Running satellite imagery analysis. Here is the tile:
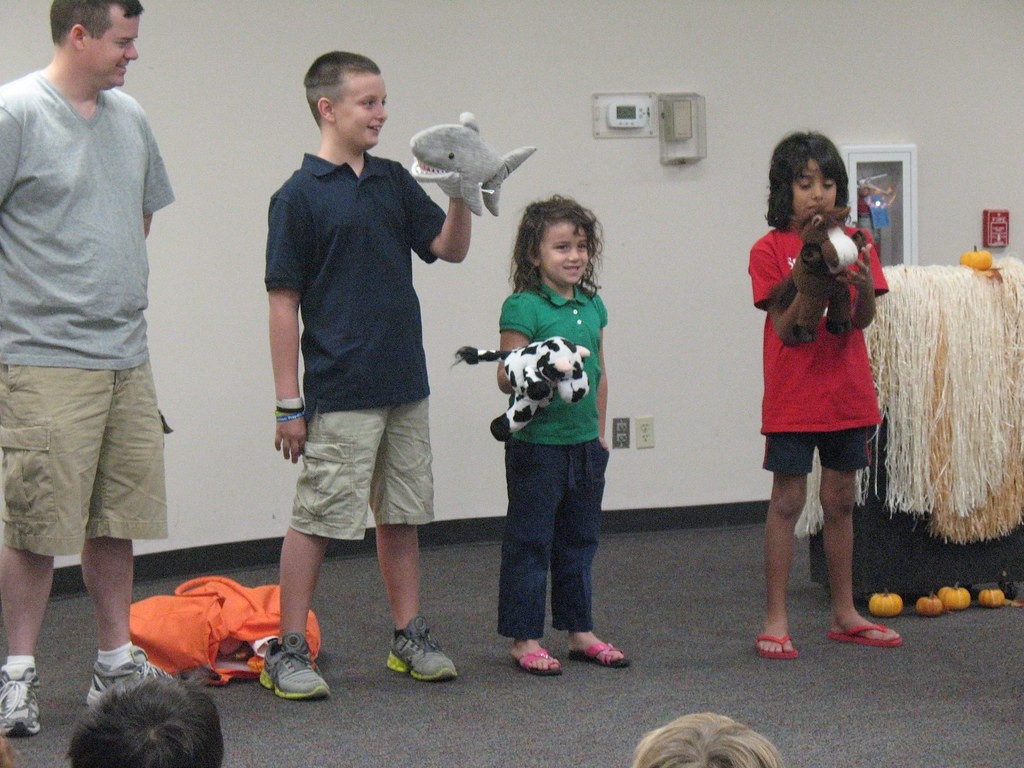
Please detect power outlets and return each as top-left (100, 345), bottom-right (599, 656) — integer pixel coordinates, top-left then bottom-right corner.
top-left (612, 418), bottom-right (631, 448)
top-left (634, 416), bottom-right (655, 447)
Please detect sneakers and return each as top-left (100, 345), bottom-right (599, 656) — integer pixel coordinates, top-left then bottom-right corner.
top-left (1, 666), bottom-right (46, 736)
top-left (262, 633), bottom-right (330, 700)
top-left (85, 657), bottom-right (172, 708)
top-left (387, 616), bottom-right (458, 680)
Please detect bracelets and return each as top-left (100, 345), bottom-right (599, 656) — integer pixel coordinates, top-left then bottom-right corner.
top-left (275, 396), bottom-right (307, 422)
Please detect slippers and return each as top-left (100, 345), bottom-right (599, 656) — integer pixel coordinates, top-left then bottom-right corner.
top-left (826, 622), bottom-right (903, 647)
top-left (569, 640), bottom-right (625, 665)
top-left (753, 634), bottom-right (801, 659)
top-left (511, 643), bottom-right (562, 674)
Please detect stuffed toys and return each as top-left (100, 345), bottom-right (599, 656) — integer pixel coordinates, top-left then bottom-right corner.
top-left (769, 205), bottom-right (868, 342)
top-left (450, 336), bottom-right (591, 442)
top-left (409, 111), bottom-right (538, 216)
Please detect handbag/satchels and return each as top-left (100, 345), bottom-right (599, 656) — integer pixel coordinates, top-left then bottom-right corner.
top-left (129, 573), bottom-right (323, 686)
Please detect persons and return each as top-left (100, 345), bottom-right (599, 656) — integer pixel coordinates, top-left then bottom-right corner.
top-left (747, 130), bottom-right (904, 659)
top-left (67, 671), bottom-right (224, 768)
top-left (258, 50), bottom-right (472, 700)
top-left (0, 0), bottom-right (177, 738)
top-left (632, 712), bottom-right (785, 768)
top-left (496, 194), bottom-right (629, 677)
